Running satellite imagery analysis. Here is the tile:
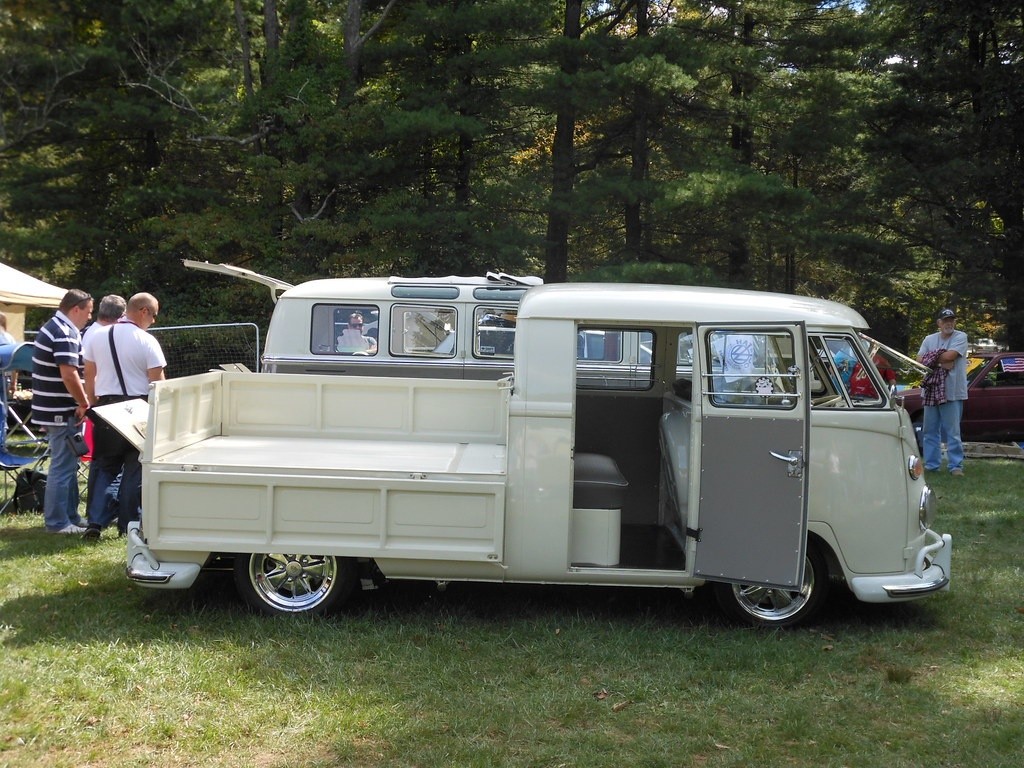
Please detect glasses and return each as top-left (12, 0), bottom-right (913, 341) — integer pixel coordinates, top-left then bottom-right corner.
top-left (73, 294), bottom-right (91, 307)
top-left (140, 307), bottom-right (158, 323)
top-left (349, 323), bottom-right (363, 328)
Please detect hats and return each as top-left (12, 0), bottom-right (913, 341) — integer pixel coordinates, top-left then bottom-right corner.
top-left (939, 309), bottom-right (956, 320)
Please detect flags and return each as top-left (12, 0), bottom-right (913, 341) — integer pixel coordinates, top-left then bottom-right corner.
top-left (1001, 357), bottom-right (1024, 372)
top-left (714, 335), bottom-right (754, 382)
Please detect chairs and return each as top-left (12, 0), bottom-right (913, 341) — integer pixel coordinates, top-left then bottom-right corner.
top-left (0, 342), bottom-right (94, 515)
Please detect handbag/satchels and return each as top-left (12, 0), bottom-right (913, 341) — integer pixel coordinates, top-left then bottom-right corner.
top-left (64, 417), bottom-right (89, 457)
top-left (13, 469), bottom-right (48, 513)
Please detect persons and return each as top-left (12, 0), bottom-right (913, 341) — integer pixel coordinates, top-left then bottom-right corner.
top-left (0, 314), bottom-right (19, 452)
top-left (338, 313), bottom-right (377, 353)
top-left (850, 352), bottom-right (896, 397)
top-left (30, 288), bottom-right (94, 533)
top-left (914, 309), bottom-right (969, 476)
top-left (80, 293), bottom-right (168, 539)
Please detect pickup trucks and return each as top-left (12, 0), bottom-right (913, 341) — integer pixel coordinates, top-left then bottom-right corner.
top-left (92, 283), bottom-right (953, 630)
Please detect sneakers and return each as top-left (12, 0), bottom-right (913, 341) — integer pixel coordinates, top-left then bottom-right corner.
top-left (47, 524), bottom-right (88, 534)
top-left (77, 517), bottom-right (89, 527)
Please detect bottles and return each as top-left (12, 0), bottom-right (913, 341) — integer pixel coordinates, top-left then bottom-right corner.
top-left (17, 383), bottom-right (22, 391)
top-left (7, 379), bottom-right (10, 387)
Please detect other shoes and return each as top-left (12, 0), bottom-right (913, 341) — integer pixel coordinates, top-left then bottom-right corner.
top-left (79, 522), bottom-right (102, 539)
top-left (117, 526), bottom-right (127, 537)
top-left (951, 469), bottom-right (964, 476)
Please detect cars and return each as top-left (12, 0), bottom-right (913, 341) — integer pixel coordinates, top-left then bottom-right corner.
top-left (895, 352), bottom-right (1024, 443)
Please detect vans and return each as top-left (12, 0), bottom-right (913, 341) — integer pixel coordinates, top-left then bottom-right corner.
top-left (183, 259), bottom-right (654, 381)
top-left (371, 307), bottom-right (652, 364)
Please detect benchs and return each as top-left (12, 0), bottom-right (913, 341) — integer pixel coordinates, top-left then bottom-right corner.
top-left (571, 451), bottom-right (631, 566)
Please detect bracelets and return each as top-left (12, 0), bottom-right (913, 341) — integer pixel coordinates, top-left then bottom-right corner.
top-left (11, 381), bottom-right (16, 384)
